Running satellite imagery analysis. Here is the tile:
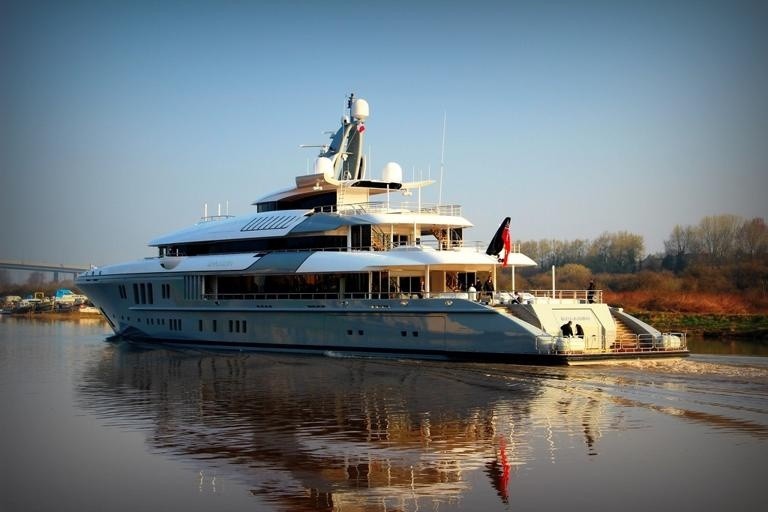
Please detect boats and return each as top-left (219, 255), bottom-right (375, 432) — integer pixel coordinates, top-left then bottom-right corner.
top-left (76, 90), bottom-right (691, 366)
top-left (0, 289), bottom-right (102, 314)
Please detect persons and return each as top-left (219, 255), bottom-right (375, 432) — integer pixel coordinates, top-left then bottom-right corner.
top-left (561, 320), bottom-right (573, 338)
top-left (473, 277), bottom-right (482, 299)
top-left (586, 279), bottom-right (594, 303)
top-left (576, 324), bottom-right (584, 339)
top-left (512, 291), bottom-right (520, 304)
top-left (483, 276), bottom-right (494, 299)
